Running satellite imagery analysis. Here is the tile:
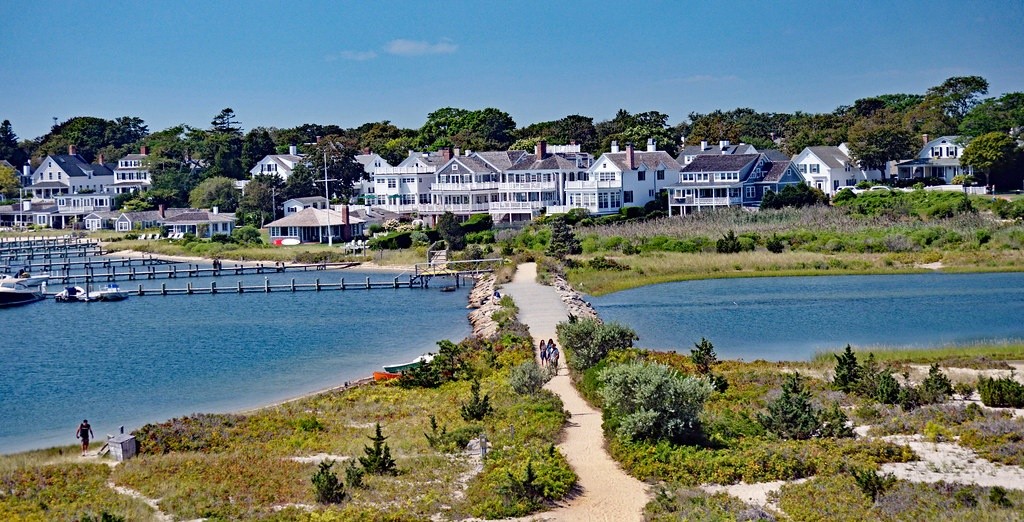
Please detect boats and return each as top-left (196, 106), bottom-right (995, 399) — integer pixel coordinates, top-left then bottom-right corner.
top-left (0, 279), bottom-right (46, 307)
top-left (55, 286), bottom-right (85, 302)
top-left (0, 274), bottom-right (50, 286)
top-left (99, 288), bottom-right (129, 300)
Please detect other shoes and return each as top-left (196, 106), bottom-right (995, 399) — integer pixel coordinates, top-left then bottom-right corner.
top-left (82, 453), bottom-right (88, 456)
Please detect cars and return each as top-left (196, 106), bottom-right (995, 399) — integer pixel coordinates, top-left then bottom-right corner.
top-left (870, 186), bottom-right (891, 191)
top-left (842, 186), bottom-right (866, 194)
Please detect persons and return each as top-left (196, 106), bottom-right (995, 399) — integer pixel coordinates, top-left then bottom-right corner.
top-left (540, 339), bottom-right (559, 376)
top-left (76, 419), bottom-right (94, 456)
top-left (493, 290), bottom-right (501, 298)
